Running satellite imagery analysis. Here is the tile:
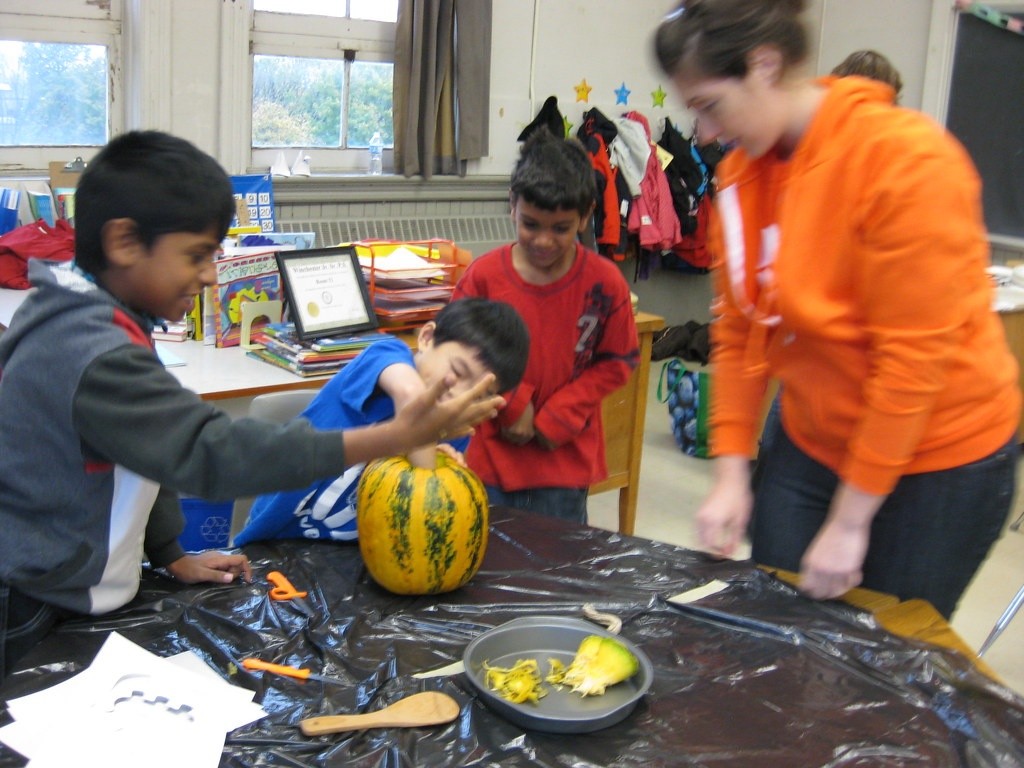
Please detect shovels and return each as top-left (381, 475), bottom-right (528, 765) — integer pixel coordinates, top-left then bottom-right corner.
top-left (300, 692), bottom-right (460, 736)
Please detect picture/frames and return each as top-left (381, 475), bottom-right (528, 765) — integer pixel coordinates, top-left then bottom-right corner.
top-left (273, 244), bottom-right (380, 339)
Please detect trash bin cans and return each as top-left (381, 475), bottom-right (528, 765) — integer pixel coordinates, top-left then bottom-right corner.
top-left (178, 496), bottom-right (235, 554)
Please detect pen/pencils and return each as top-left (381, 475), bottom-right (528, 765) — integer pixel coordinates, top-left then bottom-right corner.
top-left (164, 363), bottom-right (187, 368)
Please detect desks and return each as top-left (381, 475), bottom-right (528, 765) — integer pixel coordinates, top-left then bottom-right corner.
top-left (0, 307), bottom-right (1024, 765)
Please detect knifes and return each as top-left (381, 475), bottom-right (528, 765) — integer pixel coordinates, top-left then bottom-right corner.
top-left (243, 660), bottom-right (357, 688)
top-left (267, 571), bottom-right (314, 619)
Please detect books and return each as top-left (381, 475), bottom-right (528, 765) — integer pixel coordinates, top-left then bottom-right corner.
top-left (152, 244), bottom-right (450, 378)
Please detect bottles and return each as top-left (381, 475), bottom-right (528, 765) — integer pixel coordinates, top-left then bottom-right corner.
top-left (368, 132), bottom-right (383, 175)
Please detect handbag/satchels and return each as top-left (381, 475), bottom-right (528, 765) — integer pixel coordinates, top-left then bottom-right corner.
top-left (656, 359), bottom-right (721, 459)
top-left (0, 219), bottom-right (75, 291)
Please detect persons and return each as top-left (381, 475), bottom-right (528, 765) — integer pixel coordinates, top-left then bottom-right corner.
top-left (652, 0), bottom-right (1023, 624)
top-left (0, 129), bottom-right (507, 688)
top-left (232, 298), bottom-right (530, 546)
top-left (450, 138), bottom-right (640, 525)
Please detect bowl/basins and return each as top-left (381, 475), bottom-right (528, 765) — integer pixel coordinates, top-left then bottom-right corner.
top-left (463, 615), bottom-right (653, 732)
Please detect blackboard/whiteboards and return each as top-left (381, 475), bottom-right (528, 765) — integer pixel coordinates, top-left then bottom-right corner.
top-left (920, 0), bottom-right (1024, 254)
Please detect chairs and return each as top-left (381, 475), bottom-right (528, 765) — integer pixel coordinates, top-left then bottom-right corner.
top-left (231, 384), bottom-right (324, 550)
